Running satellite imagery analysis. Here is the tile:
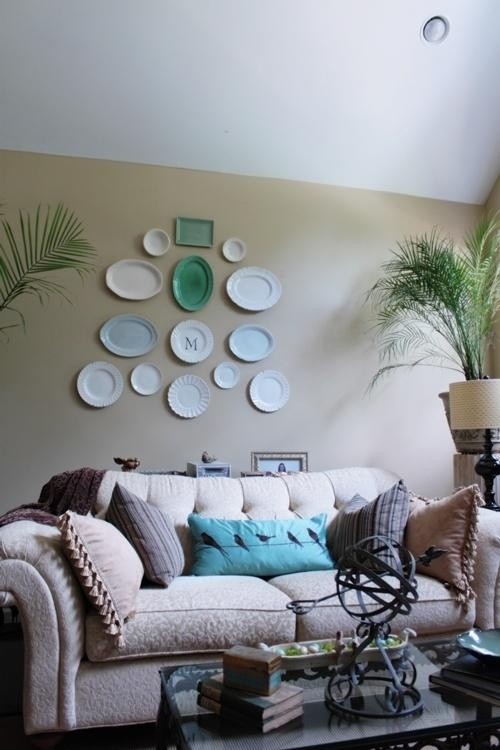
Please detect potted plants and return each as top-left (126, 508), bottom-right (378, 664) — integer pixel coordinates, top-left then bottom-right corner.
top-left (360, 208), bottom-right (500, 452)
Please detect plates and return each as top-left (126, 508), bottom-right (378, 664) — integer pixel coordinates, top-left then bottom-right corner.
top-left (169, 318), bottom-right (214, 364)
top-left (167, 374), bottom-right (210, 418)
top-left (99, 313), bottom-right (160, 358)
top-left (213, 361), bottom-right (241, 390)
top-left (130, 362), bottom-right (164, 397)
top-left (105, 258), bottom-right (164, 302)
top-left (228, 323), bottom-right (276, 363)
top-left (225, 265), bottom-right (283, 312)
top-left (249, 369), bottom-right (290, 413)
top-left (76, 360), bottom-right (124, 408)
top-left (175, 216), bottom-right (215, 248)
top-left (172, 254), bottom-right (215, 312)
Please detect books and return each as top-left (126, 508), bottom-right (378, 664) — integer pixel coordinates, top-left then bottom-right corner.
top-left (429, 655), bottom-right (500, 706)
top-left (197, 675), bottom-right (304, 734)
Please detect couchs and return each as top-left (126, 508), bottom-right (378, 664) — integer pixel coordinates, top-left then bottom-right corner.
top-left (0, 468), bottom-right (500, 737)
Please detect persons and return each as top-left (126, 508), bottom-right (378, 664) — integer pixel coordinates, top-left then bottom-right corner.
top-left (278, 463), bottom-right (286, 472)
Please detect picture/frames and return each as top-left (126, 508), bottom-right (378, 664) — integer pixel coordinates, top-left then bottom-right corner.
top-left (251, 451), bottom-right (309, 473)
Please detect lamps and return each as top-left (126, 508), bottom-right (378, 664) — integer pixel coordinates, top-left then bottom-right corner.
top-left (424, 19), bottom-right (445, 42)
top-left (449, 378), bottom-right (500, 511)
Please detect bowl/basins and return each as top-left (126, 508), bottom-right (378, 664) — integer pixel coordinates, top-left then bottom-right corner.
top-left (143, 228), bottom-right (171, 257)
top-left (456, 627), bottom-right (500, 666)
top-left (222, 238), bottom-right (247, 262)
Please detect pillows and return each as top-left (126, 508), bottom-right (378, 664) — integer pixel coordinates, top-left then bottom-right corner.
top-left (59, 511), bottom-right (146, 649)
top-left (187, 513), bottom-right (336, 575)
top-left (327, 480), bottom-right (410, 575)
top-left (106, 482), bottom-right (186, 588)
top-left (405, 484), bottom-right (486, 613)
